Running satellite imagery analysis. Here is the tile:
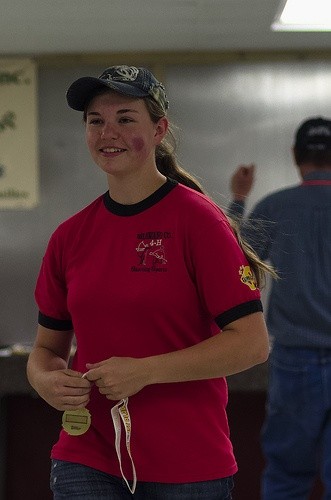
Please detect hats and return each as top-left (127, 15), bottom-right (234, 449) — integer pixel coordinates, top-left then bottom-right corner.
top-left (296, 118), bottom-right (330, 150)
top-left (65, 65), bottom-right (168, 112)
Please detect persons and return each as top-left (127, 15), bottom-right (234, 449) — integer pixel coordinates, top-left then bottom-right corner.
top-left (24, 66), bottom-right (273, 500)
top-left (222, 115), bottom-right (331, 500)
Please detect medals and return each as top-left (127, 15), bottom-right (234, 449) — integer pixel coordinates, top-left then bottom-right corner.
top-left (61, 402), bottom-right (92, 437)
